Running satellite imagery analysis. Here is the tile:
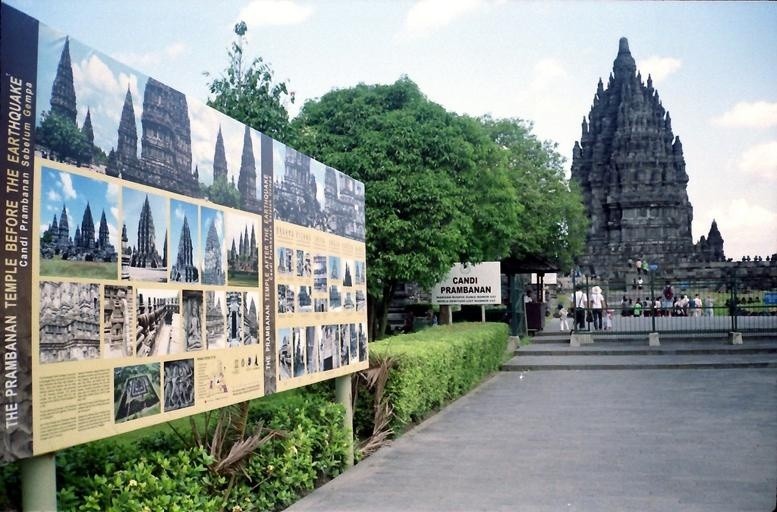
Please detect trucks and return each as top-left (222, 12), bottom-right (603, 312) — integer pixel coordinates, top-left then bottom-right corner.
top-left (385, 309), bottom-right (415, 334)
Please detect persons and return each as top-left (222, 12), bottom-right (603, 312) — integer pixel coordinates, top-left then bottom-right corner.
top-left (524, 258), bottom-right (715, 332)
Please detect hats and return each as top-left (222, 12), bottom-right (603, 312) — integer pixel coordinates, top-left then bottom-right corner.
top-left (576, 284), bottom-right (582, 289)
top-left (592, 286), bottom-right (603, 294)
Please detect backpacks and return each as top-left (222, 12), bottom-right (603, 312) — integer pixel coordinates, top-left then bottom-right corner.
top-left (664, 285), bottom-right (674, 300)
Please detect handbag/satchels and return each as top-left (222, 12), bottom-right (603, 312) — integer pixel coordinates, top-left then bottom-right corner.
top-left (575, 307), bottom-right (585, 322)
top-left (586, 311), bottom-right (595, 323)
